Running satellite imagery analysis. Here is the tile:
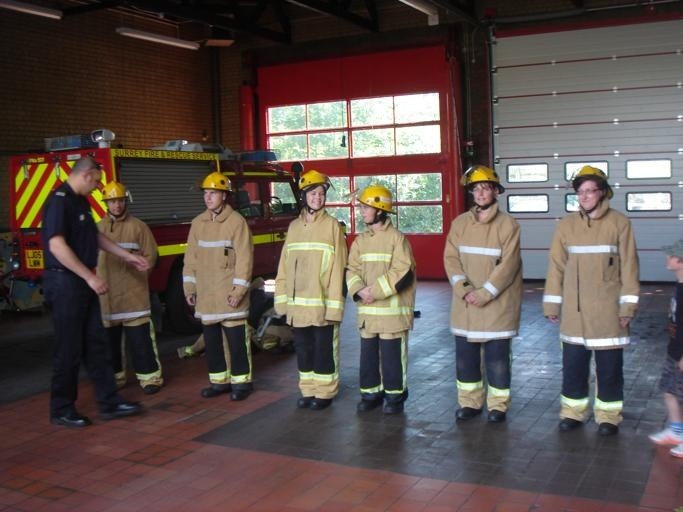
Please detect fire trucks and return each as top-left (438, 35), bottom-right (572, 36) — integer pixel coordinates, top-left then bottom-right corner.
top-left (6, 125), bottom-right (349, 338)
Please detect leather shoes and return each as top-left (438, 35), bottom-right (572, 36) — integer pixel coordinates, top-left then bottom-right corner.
top-left (49, 386), bottom-right (160, 427)
top-left (297, 397), bottom-right (314, 407)
top-left (382, 402), bottom-right (402, 414)
top-left (230, 389), bottom-right (249, 401)
top-left (358, 400), bottom-right (376, 411)
top-left (598, 423), bottom-right (618, 436)
top-left (309, 398), bottom-right (331, 409)
top-left (487, 410), bottom-right (504, 422)
top-left (455, 407), bottom-right (481, 420)
top-left (201, 384), bottom-right (231, 398)
top-left (558, 418), bottom-right (578, 430)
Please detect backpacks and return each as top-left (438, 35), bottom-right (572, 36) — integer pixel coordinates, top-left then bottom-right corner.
top-left (253, 307), bottom-right (296, 355)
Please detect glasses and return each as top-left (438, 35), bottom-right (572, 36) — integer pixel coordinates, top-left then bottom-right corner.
top-left (575, 188), bottom-right (601, 195)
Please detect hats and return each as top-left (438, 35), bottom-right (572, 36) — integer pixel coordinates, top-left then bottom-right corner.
top-left (661, 239), bottom-right (682, 258)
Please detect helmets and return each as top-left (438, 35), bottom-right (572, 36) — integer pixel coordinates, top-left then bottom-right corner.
top-left (356, 185), bottom-right (392, 213)
top-left (100, 181), bottom-right (128, 201)
top-left (298, 170), bottom-right (330, 190)
top-left (459, 165), bottom-right (504, 195)
top-left (565, 165), bottom-right (612, 199)
top-left (200, 172), bottom-right (232, 192)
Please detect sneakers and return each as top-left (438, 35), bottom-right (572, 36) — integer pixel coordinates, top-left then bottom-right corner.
top-left (669, 444), bottom-right (682, 459)
top-left (647, 429), bottom-right (682, 445)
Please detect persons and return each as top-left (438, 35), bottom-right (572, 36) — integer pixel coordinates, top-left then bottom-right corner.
top-left (442, 164), bottom-right (524, 424)
top-left (344, 184), bottom-right (416, 417)
top-left (95, 177), bottom-right (165, 395)
top-left (181, 171), bottom-right (254, 402)
top-left (272, 169), bottom-right (347, 410)
top-left (541, 164), bottom-right (641, 437)
top-left (40, 154), bottom-right (151, 428)
top-left (646, 239), bottom-right (682, 458)
